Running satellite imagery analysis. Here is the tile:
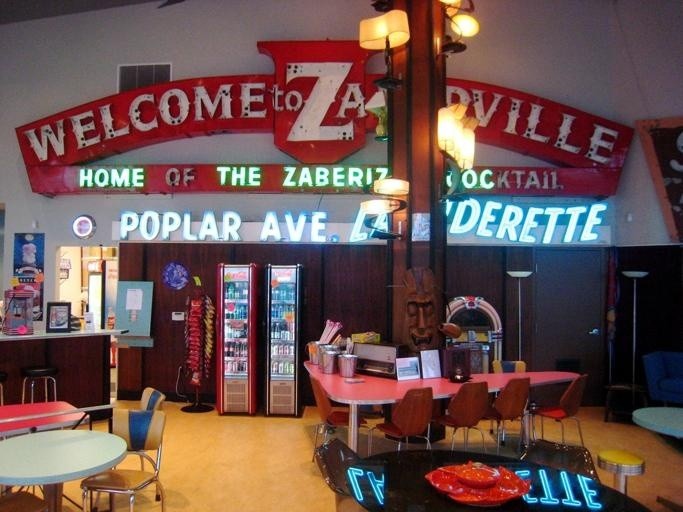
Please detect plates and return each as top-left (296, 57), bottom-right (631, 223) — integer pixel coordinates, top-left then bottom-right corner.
top-left (424, 461), bottom-right (530, 507)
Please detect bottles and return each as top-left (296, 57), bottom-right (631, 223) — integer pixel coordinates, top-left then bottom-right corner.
top-left (271, 321), bottom-right (294, 341)
top-left (224, 340), bottom-right (246, 357)
top-left (107, 308), bottom-right (114, 329)
top-left (270, 343), bottom-right (293, 355)
top-left (271, 287), bottom-right (295, 300)
top-left (270, 360), bottom-right (294, 375)
top-left (224, 323), bottom-right (246, 338)
top-left (224, 303), bottom-right (247, 319)
top-left (270, 303), bottom-right (294, 318)
top-left (224, 359), bottom-right (247, 372)
top-left (224, 283), bottom-right (247, 299)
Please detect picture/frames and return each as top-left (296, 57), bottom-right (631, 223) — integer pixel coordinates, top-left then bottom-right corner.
top-left (46, 302), bottom-right (71, 332)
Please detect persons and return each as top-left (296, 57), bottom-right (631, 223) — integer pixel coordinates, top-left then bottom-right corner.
top-left (394, 267), bottom-right (442, 353)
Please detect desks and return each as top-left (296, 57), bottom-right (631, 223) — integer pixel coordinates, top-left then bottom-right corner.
top-left (0, 430), bottom-right (127, 512)
top-left (343, 448), bottom-right (650, 511)
top-left (0, 401), bottom-right (90, 441)
top-left (305, 361), bottom-right (583, 456)
top-left (632, 407), bottom-right (683, 512)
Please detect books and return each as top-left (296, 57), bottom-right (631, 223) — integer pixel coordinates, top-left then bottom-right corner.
top-left (395, 356), bottom-right (420, 382)
top-left (418, 348), bottom-right (442, 380)
top-left (442, 348), bottom-right (474, 379)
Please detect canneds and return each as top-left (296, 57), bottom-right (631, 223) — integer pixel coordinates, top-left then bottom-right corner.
top-left (269, 289), bottom-right (296, 375)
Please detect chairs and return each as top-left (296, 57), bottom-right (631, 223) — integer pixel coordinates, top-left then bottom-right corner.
top-left (314, 437), bottom-right (360, 499)
top-left (93, 387), bottom-right (166, 512)
top-left (533, 373), bottom-right (586, 448)
top-left (309, 374), bottom-right (370, 462)
top-left (644, 351), bottom-right (683, 402)
top-left (80, 410), bottom-right (166, 512)
top-left (427, 381), bottom-right (489, 451)
top-left (465, 377), bottom-right (531, 442)
top-left (369, 386), bottom-right (433, 453)
top-left (519, 438), bottom-right (597, 481)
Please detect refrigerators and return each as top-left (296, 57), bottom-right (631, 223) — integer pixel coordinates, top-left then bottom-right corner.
top-left (214, 263), bottom-right (258, 416)
top-left (261, 264), bottom-right (307, 418)
top-left (87, 257), bottom-right (118, 368)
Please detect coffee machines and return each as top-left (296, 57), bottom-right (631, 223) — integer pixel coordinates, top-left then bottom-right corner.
top-left (449, 347), bottom-right (470, 381)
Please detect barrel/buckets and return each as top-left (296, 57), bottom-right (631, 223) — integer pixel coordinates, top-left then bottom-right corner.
top-left (304, 341), bottom-right (327, 365)
top-left (318, 344), bottom-right (340, 368)
top-left (337, 354), bottom-right (358, 377)
top-left (322, 351), bottom-right (337, 373)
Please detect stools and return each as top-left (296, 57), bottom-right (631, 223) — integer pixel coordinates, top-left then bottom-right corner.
top-left (604, 386), bottom-right (633, 424)
top-left (22, 365), bottom-right (57, 404)
top-left (0, 371), bottom-right (8, 406)
top-left (598, 451), bottom-right (645, 496)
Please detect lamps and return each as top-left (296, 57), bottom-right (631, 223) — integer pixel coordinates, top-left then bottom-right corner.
top-left (437, 104), bottom-right (480, 170)
top-left (359, 9), bottom-right (411, 91)
top-left (506, 271), bottom-right (533, 361)
top-left (622, 271), bottom-right (649, 389)
top-left (360, 179), bottom-right (409, 240)
top-left (441, 1), bottom-right (480, 53)
